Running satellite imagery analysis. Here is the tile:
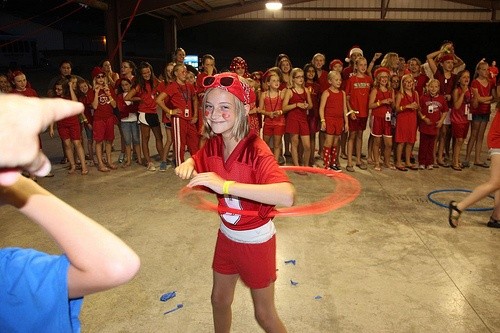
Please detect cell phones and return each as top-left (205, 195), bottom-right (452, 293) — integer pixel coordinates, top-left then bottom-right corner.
top-left (185, 108), bottom-right (189, 117)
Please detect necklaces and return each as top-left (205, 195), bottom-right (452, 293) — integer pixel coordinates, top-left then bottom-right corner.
top-left (405, 90), bottom-right (415, 104)
top-left (269, 90), bottom-right (279, 112)
top-left (293, 86), bottom-right (306, 102)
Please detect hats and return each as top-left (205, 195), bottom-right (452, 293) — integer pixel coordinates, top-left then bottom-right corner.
top-left (329, 59), bottom-right (344, 71)
top-left (373, 67), bottom-right (390, 79)
top-left (439, 53), bottom-right (454, 63)
top-left (345, 45), bottom-right (363, 63)
top-left (229, 56), bottom-right (247, 78)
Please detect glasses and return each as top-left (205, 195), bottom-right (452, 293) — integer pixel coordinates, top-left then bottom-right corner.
top-left (235, 65), bottom-right (246, 70)
top-left (96, 74), bottom-right (105, 78)
top-left (292, 75), bottom-right (305, 80)
top-left (202, 75), bottom-right (237, 88)
top-left (54, 88), bottom-right (63, 92)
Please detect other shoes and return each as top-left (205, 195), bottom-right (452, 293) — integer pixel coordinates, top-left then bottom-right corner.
top-left (23, 150), bottom-right (176, 181)
top-left (276, 147), bottom-right (491, 175)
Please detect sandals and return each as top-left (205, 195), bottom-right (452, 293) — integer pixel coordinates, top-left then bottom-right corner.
top-left (487, 216), bottom-right (500, 228)
top-left (449, 200), bottom-right (462, 228)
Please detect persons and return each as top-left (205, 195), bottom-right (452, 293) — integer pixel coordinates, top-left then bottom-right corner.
top-left (46, 59), bottom-right (119, 174)
top-left (0, 70), bottom-right (55, 177)
top-left (175, 72), bottom-right (296, 333)
top-left (115, 60), bottom-right (171, 171)
top-left (451, 58), bottom-right (500, 171)
top-left (449, 104), bottom-right (500, 228)
top-left (257, 53), bottom-right (330, 175)
top-left (0, 93), bottom-right (140, 333)
top-left (319, 42), bottom-right (465, 173)
top-left (155, 47), bottom-right (262, 169)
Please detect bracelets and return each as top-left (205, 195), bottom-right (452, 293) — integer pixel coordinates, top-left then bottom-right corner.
top-left (421, 115), bottom-right (425, 120)
top-left (83, 119), bottom-right (89, 125)
top-left (296, 103), bottom-right (297, 107)
top-left (321, 119), bottom-right (325, 123)
top-left (377, 101), bottom-right (380, 105)
top-left (224, 179), bottom-right (236, 195)
top-left (169, 110), bottom-right (172, 115)
top-left (400, 106), bottom-right (403, 110)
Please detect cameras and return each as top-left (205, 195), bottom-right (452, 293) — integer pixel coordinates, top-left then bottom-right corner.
top-left (428, 106), bottom-right (433, 113)
top-left (385, 113), bottom-right (392, 121)
